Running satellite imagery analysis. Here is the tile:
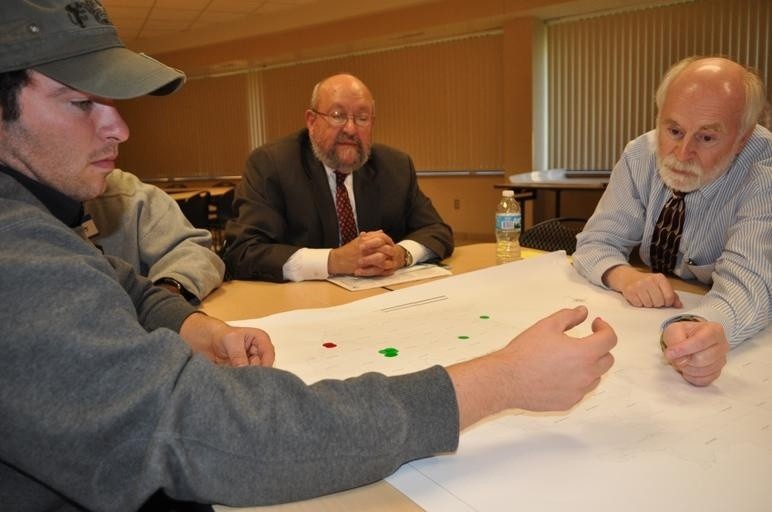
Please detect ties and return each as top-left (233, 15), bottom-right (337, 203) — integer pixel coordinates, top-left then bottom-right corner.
top-left (650, 190), bottom-right (685, 276)
top-left (335, 172), bottom-right (358, 246)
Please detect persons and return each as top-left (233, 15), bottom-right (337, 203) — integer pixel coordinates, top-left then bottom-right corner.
top-left (572, 57), bottom-right (771, 386)
top-left (224, 74), bottom-right (453, 284)
top-left (80, 162), bottom-right (227, 306)
top-left (1, 0), bottom-right (618, 512)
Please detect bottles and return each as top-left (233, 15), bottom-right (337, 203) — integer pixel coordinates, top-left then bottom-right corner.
top-left (494, 190), bottom-right (521, 267)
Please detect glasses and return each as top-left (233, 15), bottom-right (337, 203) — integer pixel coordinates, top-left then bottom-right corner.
top-left (310, 108), bottom-right (373, 126)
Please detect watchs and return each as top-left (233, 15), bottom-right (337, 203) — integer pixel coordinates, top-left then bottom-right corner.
top-left (404, 251), bottom-right (411, 266)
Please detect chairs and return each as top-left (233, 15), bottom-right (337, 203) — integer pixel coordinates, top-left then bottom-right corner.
top-left (519, 216), bottom-right (591, 255)
top-left (163, 182), bottom-right (235, 252)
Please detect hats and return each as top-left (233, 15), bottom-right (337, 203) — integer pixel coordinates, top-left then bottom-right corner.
top-left (0, 0), bottom-right (187, 100)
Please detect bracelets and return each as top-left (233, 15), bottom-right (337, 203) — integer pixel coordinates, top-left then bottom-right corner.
top-left (160, 278), bottom-right (182, 292)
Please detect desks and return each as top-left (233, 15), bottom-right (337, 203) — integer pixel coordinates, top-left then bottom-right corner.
top-left (194, 242), bottom-right (771, 511)
top-left (493, 177), bottom-right (612, 237)
